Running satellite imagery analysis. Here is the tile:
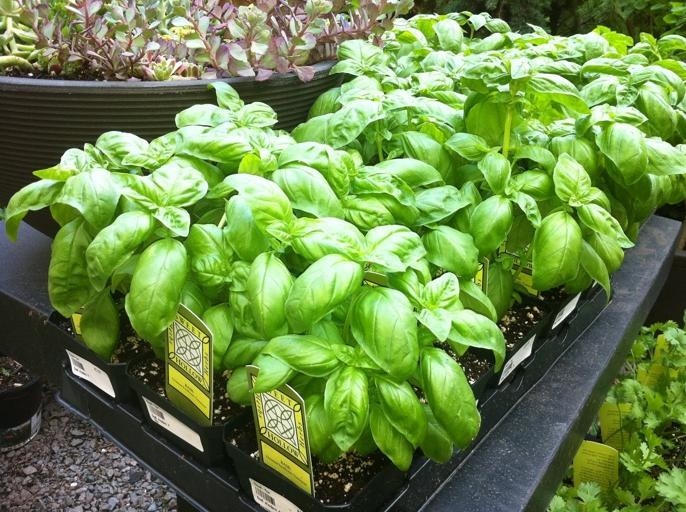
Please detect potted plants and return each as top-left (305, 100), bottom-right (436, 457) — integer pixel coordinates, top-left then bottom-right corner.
top-left (0, 1), bottom-right (684, 512)
